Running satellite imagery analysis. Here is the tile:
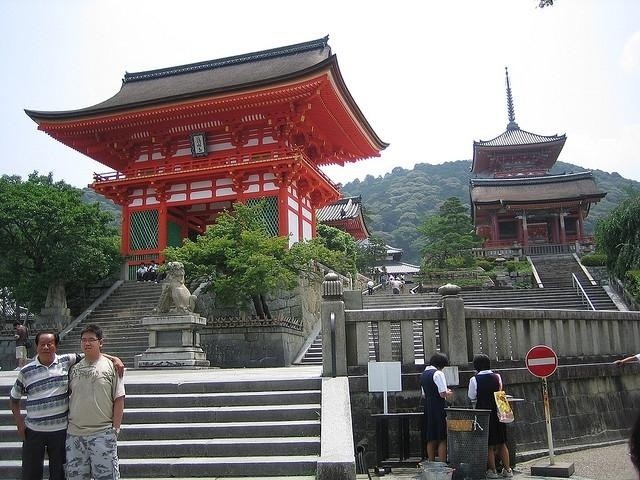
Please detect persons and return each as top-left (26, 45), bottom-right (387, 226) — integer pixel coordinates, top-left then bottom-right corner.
top-left (12, 319), bottom-right (30, 373)
top-left (134, 260), bottom-right (167, 284)
top-left (61, 325), bottom-right (127, 480)
top-left (9, 328), bottom-right (126, 480)
top-left (366, 279), bottom-right (375, 295)
top-left (374, 264), bottom-right (406, 294)
top-left (466, 353), bottom-right (515, 479)
top-left (612, 353), bottom-right (640, 367)
top-left (419, 351), bottom-right (454, 464)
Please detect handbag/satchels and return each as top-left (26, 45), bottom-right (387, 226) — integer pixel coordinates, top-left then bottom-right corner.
top-left (493, 389), bottom-right (516, 424)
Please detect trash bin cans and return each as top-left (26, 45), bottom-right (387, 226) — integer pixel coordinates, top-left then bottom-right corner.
top-left (444, 408), bottom-right (492, 480)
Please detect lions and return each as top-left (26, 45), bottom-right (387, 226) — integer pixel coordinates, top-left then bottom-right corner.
top-left (153, 262), bottom-right (198, 316)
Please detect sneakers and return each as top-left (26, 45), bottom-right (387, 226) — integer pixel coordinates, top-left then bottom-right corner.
top-left (486, 468), bottom-right (499, 479)
top-left (499, 468), bottom-right (514, 477)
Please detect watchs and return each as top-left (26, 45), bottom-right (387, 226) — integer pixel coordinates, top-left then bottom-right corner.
top-left (112, 427), bottom-right (121, 435)
